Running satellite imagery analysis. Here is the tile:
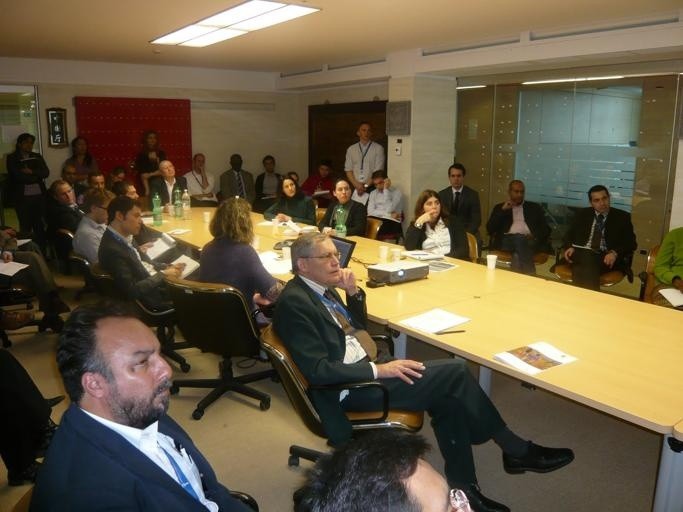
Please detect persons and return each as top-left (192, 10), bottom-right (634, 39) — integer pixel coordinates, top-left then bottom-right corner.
top-left (344, 120), bottom-right (385, 206)
top-left (561, 184), bottom-right (639, 292)
top-left (220, 153), bottom-right (315, 225)
top-left (271, 229), bottom-right (577, 512)
top-left (486, 179), bottom-right (552, 275)
top-left (48, 164), bottom-right (153, 270)
top-left (199, 197), bottom-right (284, 361)
top-left (403, 163), bottom-right (481, 259)
top-left (288, 428), bottom-right (471, 511)
top-left (0, 207), bottom-right (73, 330)
top-left (64, 136), bottom-right (102, 178)
top-left (649, 226), bottom-right (683, 311)
top-left (300, 160), bottom-right (401, 238)
top-left (133, 128), bottom-right (166, 194)
top-left (0, 346), bottom-right (62, 486)
top-left (149, 153), bottom-right (220, 208)
top-left (26, 298), bottom-right (261, 512)
top-left (5, 133), bottom-right (50, 233)
top-left (98, 196), bottom-right (186, 310)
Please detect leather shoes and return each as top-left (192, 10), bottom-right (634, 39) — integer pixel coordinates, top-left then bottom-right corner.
top-left (502, 440), bottom-right (575, 476)
top-left (8, 460), bottom-right (42, 487)
top-left (456, 482), bottom-right (512, 511)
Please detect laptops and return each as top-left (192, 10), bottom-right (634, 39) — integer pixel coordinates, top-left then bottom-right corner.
top-left (330, 235), bottom-right (357, 268)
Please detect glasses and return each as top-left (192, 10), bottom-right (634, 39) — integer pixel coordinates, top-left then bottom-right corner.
top-left (447, 485), bottom-right (473, 512)
top-left (298, 250), bottom-right (341, 261)
top-left (116, 175), bottom-right (124, 181)
top-left (94, 204), bottom-right (109, 212)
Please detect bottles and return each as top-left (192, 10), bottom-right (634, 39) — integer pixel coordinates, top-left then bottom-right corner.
top-left (335, 206), bottom-right (346, 237)
top-left (151, 193), bottom-right (162, 226)
top-left (173, 188), bottom-right (190, 219)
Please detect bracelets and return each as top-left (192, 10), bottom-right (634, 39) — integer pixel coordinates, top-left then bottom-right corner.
top-left (609, 249), bottom-right (619, 257)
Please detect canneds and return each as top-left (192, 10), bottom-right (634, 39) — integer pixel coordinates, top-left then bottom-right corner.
top-left (335, 224), bottom-right (346, 238)
top-left (174, 201), bottom-right (182, 217)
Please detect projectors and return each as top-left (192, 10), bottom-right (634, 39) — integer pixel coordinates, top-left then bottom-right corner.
top-left (365, 263), bottom-right (429, 288)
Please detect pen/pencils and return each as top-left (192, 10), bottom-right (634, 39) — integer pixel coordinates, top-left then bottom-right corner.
top-left (435, 330), bottom-right (464, 334)
top-left (411, 253), bottom-right (429, 256)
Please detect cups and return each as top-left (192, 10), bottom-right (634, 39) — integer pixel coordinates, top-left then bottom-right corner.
top-left (380, 246), bottom-right (388, 258)
top-left (272, 218), bottom-right (278, 226)
top-left (204, 212), bottom-right (210, 222)
top-left (281, 247), bottom-right (291, 259)
top-left (487, 254), bottom-right (497, 270)
top-left (391, 249), bottom-right (401, 262)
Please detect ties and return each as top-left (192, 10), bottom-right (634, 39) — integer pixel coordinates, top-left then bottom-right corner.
top-left (236, 172), bottom-right (244, 198)
top-left (159, 445), bottom-right (199, 502)
top-left (590, 214), bottom-right (603, 248)
top-left (453, 191), bottom-right (459, 217)
